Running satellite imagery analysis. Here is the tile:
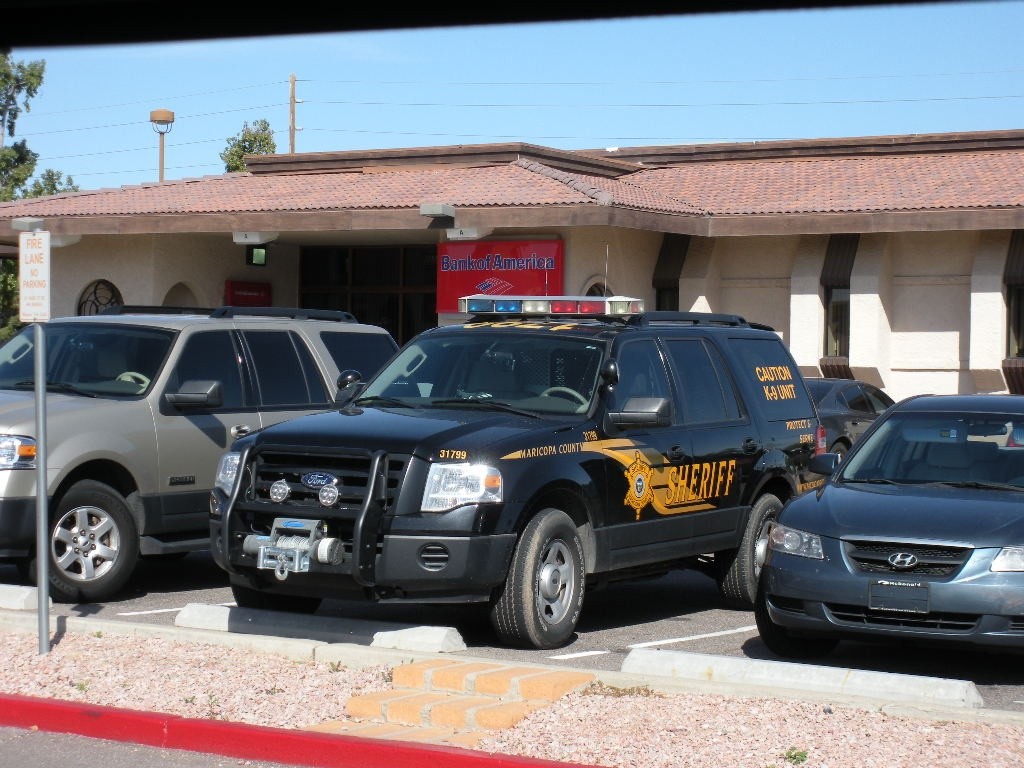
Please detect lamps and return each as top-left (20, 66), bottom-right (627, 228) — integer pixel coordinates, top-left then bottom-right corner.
top-left (419, 203), bottom-right (455, 217)
top-left (246, 244), bottom-right (267, 266)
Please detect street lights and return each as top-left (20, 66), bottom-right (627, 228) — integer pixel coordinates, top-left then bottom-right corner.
top-left (148, 107), bottom-right (176, 182)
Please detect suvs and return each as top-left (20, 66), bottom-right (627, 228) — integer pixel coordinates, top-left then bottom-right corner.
top-left (0, 304), bottom-right (424, 603)
top-left (211, 293), bottom-right (830, 648)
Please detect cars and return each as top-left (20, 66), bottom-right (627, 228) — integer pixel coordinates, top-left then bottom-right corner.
top-left (758, 394), bottom-right (1024, 659)
top-left (800, 375), bottom-right (899, 466)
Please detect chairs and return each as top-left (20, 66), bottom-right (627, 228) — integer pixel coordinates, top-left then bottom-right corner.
top-left (186, 356), bottom-right (238, 406)
top-left (907, 436), bottom-right (971, 483)
top-left (619, 369), bottom-right (693, 415)
